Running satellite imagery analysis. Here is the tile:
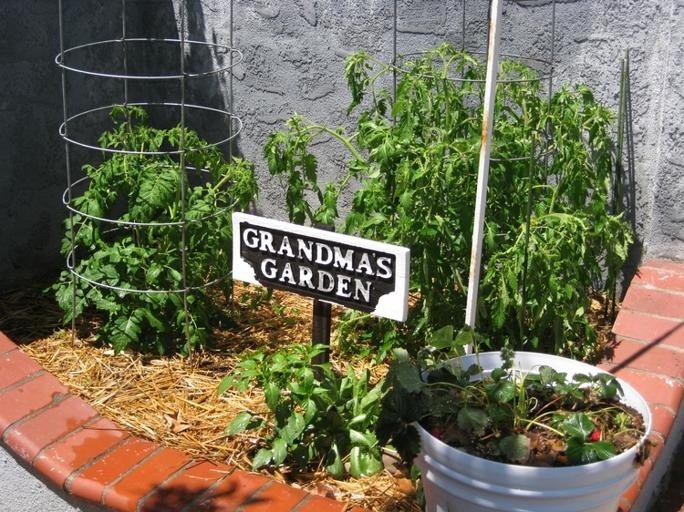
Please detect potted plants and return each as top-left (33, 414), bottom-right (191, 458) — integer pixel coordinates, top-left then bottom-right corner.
top-left (373, 324), bottom-right (654, 512)
